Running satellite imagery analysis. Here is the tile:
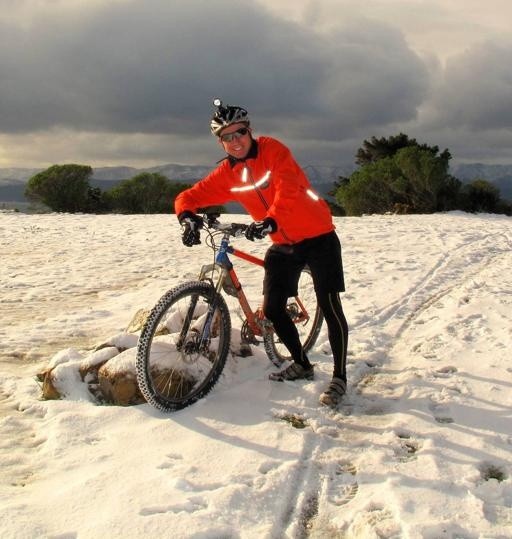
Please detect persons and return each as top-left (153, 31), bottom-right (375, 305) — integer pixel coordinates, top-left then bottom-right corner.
top-left (175, 106), bottom-right (350, 405)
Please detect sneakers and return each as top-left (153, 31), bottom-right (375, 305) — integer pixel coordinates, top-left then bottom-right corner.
top-left (318, 378), bottom-right (347, 407)
top-left (269, 362), bottom-right (314, 381)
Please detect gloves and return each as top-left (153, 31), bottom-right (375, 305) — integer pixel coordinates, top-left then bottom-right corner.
top-left (244, 220), bottom-right (273, 242)
top-left (180, 216), bottom-right (201, 247)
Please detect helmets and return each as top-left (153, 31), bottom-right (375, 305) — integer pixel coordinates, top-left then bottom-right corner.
top-left (209, 105), bottom-right (249, 136)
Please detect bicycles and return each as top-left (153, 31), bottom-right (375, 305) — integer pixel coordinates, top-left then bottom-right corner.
top-left (133, 213), bottom-right (325, 414)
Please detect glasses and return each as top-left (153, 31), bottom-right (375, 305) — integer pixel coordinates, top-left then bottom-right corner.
top-left (216, 125), bottom-right (249, 142)
